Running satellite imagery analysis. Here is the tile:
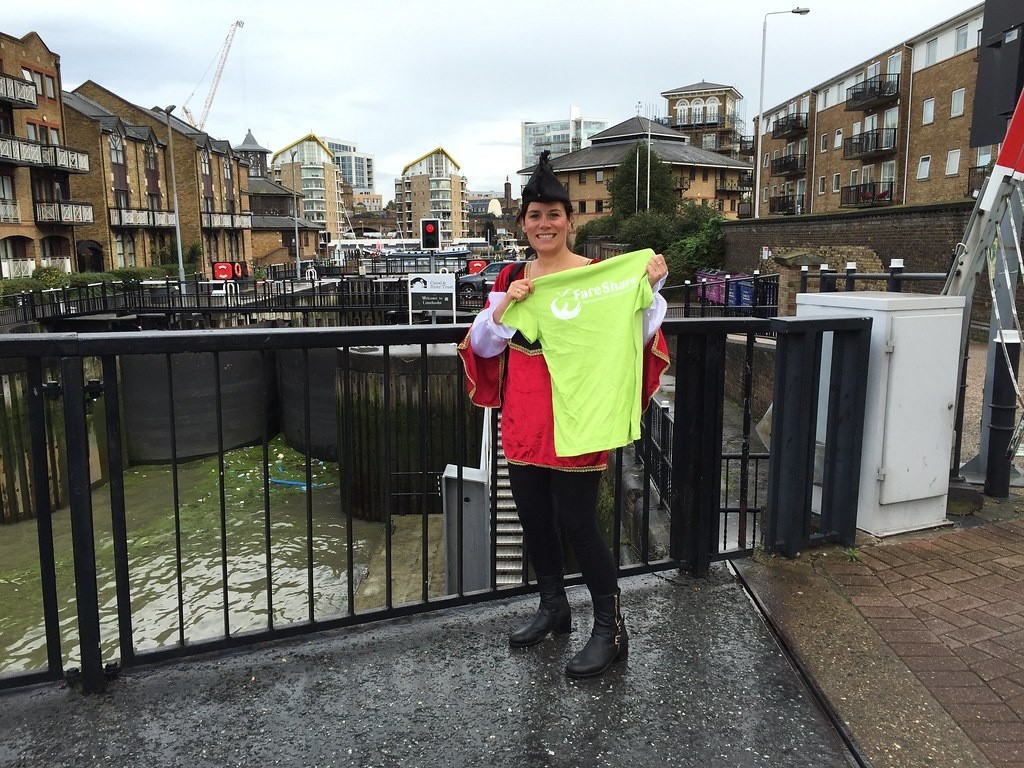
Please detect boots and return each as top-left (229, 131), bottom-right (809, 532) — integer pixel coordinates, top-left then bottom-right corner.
top-left (510, 572), bottom-right (572, 646)
top-left (564, 589), bottom-right (628, 676)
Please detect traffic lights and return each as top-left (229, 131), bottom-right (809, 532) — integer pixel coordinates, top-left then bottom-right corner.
top-left (420, 219), bottom-right (441, 251)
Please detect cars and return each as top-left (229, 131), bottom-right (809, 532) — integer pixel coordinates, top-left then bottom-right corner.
top-left (458, 262), bottom-right (514, 300)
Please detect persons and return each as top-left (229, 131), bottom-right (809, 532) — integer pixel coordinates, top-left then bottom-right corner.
top-left (469, 151), bottom-right (667, 677)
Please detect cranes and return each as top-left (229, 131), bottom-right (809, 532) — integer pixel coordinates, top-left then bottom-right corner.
top-left (182, 19), bottom-right (244, 132)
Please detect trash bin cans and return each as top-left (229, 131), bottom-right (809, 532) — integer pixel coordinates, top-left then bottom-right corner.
top-left (695, 266), bottom-right (779, 316)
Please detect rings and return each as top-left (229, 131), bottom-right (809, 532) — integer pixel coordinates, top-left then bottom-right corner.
top-left (657, 261), bottom-right (661, 265)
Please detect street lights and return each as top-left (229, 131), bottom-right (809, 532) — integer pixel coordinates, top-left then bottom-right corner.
top-left (290, 150), bottom-right (301, 281)
top-left (755, 7), bottom-right (811, 219)
top-left (151, 104), bottom-right (187, 308)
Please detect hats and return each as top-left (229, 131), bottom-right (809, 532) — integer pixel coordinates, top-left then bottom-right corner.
top-left (522, 154), bottom-right (568, 203)
